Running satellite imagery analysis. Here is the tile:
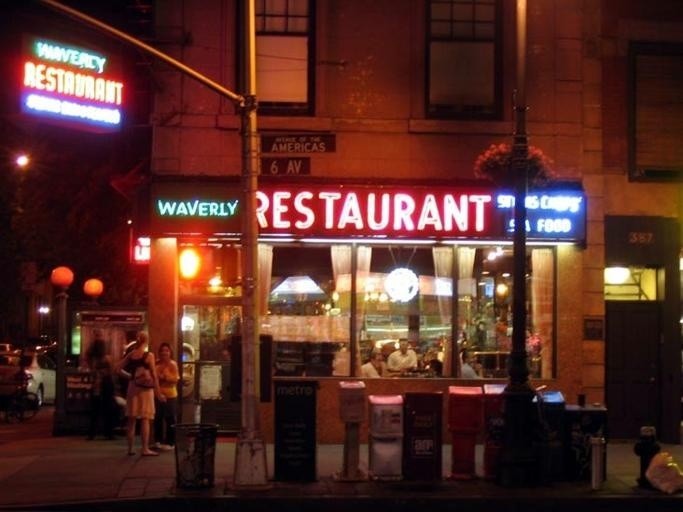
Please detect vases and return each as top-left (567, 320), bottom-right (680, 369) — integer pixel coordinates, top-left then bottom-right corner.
top-left (485, 164), bottom-right (540, 189)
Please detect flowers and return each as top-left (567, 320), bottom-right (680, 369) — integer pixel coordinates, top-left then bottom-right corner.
top-left (473, 142), bottom-right (556, 187)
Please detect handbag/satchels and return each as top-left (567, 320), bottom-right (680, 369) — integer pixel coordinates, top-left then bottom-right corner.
top-left (134, 367), bottom-right (155, 388)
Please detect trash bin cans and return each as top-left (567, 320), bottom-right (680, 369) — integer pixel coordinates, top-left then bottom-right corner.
top-left (170, 423), bottom-right (220, 492)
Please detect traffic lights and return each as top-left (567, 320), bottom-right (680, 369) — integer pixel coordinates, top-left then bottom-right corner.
top-left (177, 242), bottom-right (216, 287)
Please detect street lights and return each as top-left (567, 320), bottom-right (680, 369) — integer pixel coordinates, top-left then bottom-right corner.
top-left (50, 265), bottom-right (73, 438)
top-left (81, 278), bottom-right (103, 308)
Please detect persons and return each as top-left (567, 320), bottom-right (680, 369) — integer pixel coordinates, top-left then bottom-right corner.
top-left (83, 354), bottom-right (118, 441)
top-left (459, 350), bottom-right (483, 380)
top-left (361, 350), bottom-right (385, 379)
top-left (114, 331), bottom-right (159, 457)
top-left (387, 338), bottom-right (418, 372)
top-left (149, 342), bottom-right (181, 451)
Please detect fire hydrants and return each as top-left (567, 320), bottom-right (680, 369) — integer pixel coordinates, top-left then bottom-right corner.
top-left (633, 426), bottom-right (661, 489)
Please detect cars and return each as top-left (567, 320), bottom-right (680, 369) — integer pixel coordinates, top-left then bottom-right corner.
top-left (0, 343), bottom-right (17, 352)
top-left (26, 333), bottom-right (56, 352)
top-left (0, 350), bottom-right (55, 408)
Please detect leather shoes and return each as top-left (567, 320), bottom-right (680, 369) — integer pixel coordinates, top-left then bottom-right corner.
top-left (127, 442), bottom-right (176, 457)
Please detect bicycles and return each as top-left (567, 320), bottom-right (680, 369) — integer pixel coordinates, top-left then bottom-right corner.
top-left (0, 373), bottom-right (38, 425)
